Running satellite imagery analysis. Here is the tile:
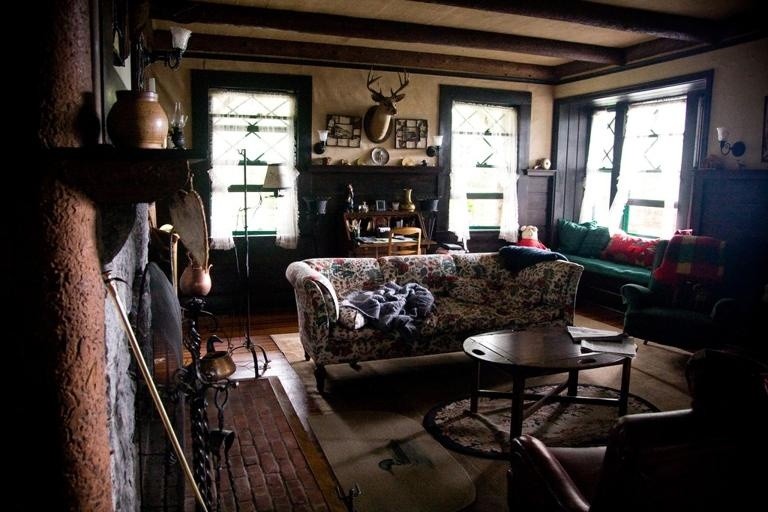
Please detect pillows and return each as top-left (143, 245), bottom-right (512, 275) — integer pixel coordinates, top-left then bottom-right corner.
top-left (579, 227), bottom-right (610, 259)
top-left (441, 269), bottom-right (489, 306)
top-left (601, 234), bottom-right (654, 269)
top-left (556, 219), bottom-right (592, 254)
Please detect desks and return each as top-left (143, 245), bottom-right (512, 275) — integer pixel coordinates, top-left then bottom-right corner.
top-left (354, 234), bottom-right (437, 260)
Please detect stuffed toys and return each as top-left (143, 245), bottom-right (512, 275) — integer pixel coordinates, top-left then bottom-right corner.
top-left (516, 225), bottom-right (547, 251)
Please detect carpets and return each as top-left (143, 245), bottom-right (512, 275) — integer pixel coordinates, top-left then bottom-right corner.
top-left (304, 408), bottom-right (478, 512)
top-left (269, 310), bottom-right (697, 506)
top-left (422, 379), bottom-right (663, 463)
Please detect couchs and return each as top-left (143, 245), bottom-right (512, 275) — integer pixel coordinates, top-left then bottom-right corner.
top-left (555, 219), bottom-right (654, 316)
top-left (619, 234), bottom-right (740, 352)
top-left (505, 406), bottom-right (717, 512)
top-left (283, 247), bottom-right (585, 397)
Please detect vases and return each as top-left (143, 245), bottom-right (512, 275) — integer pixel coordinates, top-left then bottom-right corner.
top-left (107, 88), bottom-right (170, 149)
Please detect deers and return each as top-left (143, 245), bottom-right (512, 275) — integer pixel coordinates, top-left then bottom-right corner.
top-left (365, 65), bottom-right (410, 141)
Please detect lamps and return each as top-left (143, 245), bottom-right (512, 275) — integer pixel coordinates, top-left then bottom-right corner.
top-left (133, 20), bottom-right (192, 93)
top-left (720, 128), bottom-right (746, 157)
top-left (265, 163), bottom-right (293, 196)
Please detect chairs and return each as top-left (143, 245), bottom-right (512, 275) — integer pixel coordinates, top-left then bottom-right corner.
top-left (388, 226), bottom-right (422, 255)
